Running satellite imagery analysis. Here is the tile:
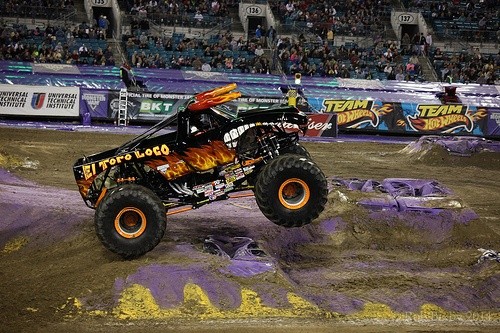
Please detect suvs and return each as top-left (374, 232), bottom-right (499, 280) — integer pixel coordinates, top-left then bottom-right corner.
top-left (73, 84), bottom-right (329, 261)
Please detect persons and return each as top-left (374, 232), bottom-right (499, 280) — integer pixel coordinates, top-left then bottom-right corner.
top-left (1, 0), bottom-right (498, 85)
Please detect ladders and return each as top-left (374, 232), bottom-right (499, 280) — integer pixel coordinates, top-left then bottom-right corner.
top-left (118, 89), bottom-right (128, 127)
